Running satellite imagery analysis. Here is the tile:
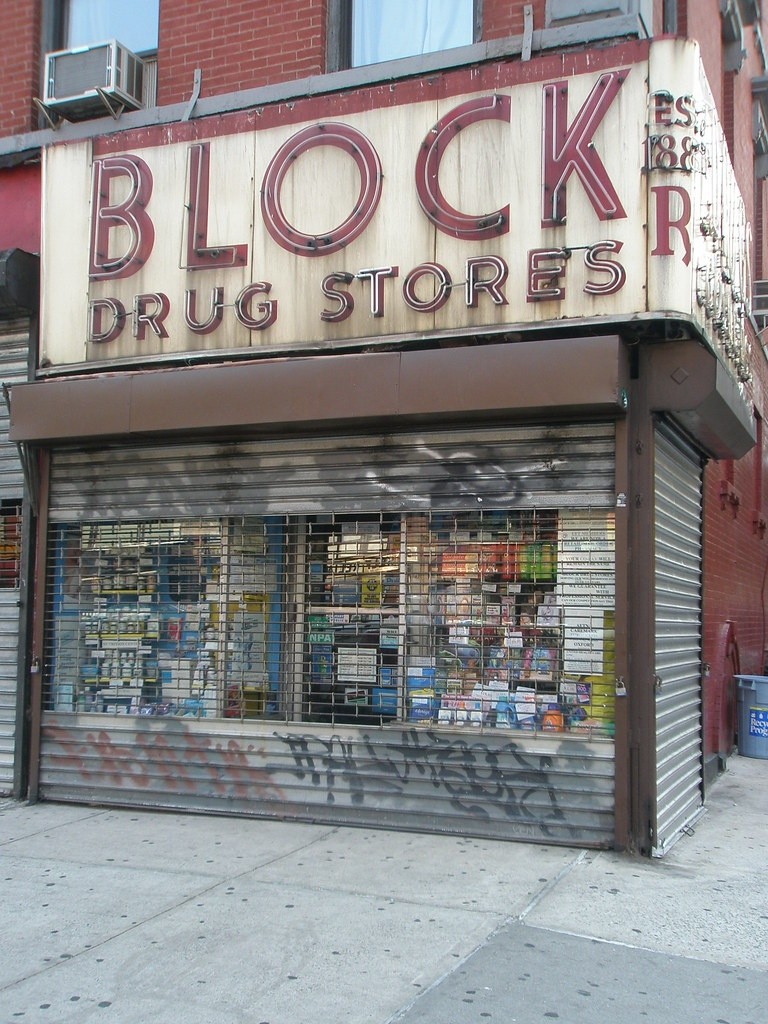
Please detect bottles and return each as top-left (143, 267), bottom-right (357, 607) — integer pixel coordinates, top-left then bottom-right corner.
top-left (85, 687), bottom-right (93, 711)
top-left (90, 702), bottom-right (96, 711)
top-left (470, 703), bottom-right (483, 728)
top-left (77, 691), bottom-right (85, 712)
top-left (497, 696), bottom-right (516, 729)
top-left (454, 703), bottom-right (468, 725)
top-left (438, 702), bottom-right (452, 724)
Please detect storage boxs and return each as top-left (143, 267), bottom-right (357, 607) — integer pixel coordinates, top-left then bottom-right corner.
top-left (79, 508), bottom-right (617, 736)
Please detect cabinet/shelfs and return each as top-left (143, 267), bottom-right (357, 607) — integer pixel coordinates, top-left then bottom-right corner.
top-left (84, 587), bottom-right (159, 683)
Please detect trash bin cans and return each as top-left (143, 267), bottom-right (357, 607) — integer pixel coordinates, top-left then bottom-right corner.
top-left (732, 674), bottom-right (768, 763)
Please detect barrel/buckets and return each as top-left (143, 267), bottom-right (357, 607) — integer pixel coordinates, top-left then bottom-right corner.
top-left (736, 674), bottom-right (768, 759)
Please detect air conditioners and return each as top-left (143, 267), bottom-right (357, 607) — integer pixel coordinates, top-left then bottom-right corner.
top-left (43, 39), bottom-right (147, 124)
top-left (752, 280), bottom-right (768, 310)
